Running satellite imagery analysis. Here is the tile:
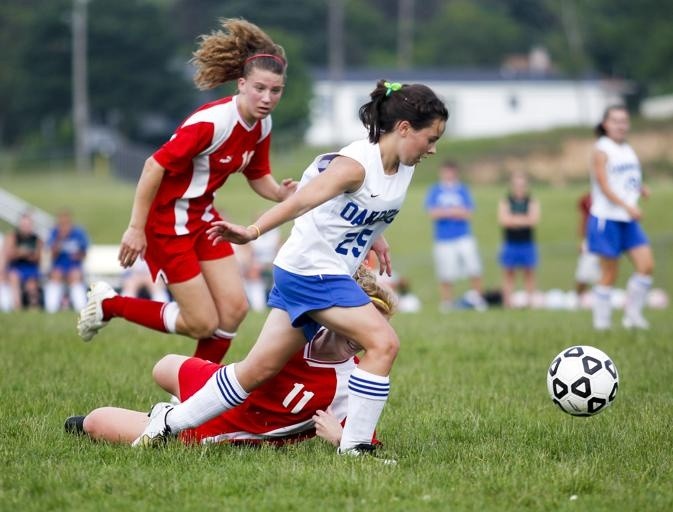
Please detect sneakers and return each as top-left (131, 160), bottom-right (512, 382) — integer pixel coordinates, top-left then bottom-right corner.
top-left (131, 402), bottom-right (175, 449)
top-left (64, 416), bottom-right (86, 436)
top-left (76, 280), bottom-right (120, 342)
top-left (336, 444), bottom-right (398, 466)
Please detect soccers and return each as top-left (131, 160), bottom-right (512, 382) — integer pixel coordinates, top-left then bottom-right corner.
top-left (548, 345), bottom-right (620, 415)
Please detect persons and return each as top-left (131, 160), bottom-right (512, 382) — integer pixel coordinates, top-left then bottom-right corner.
top-left (63, 264), bottom-right (397, 449)
top-left (77, 19), bottom-right (301, 363)
top-left (131, 79), bottom-right (450, 465)
top-left (423, 106), bottom-right (655, 331)
top-left (0, 211), bottom-right (286, 312)
